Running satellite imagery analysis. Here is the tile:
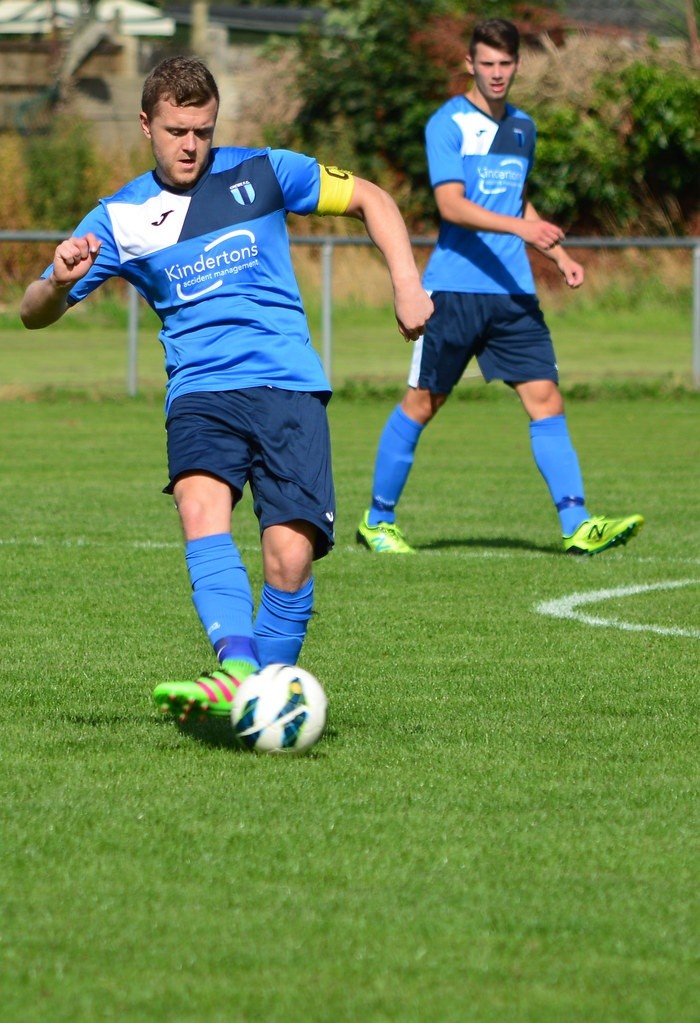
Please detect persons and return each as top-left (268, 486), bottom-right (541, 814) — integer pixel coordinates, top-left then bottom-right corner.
top-left (354, 15), bottom-right (645, 558)
top-left (19, 57), bottom-right (435, 722)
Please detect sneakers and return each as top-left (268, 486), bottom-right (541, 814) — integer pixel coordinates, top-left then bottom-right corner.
top-left (561, 515), bottom-right (644, 557)
top-left (151, 661), bottom-right (258, 723)
top-left (356, 510), bottom-right (417, 554)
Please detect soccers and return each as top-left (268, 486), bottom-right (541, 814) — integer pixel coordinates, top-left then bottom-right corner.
top-left (231, 664), bottom-right (330, 755)
